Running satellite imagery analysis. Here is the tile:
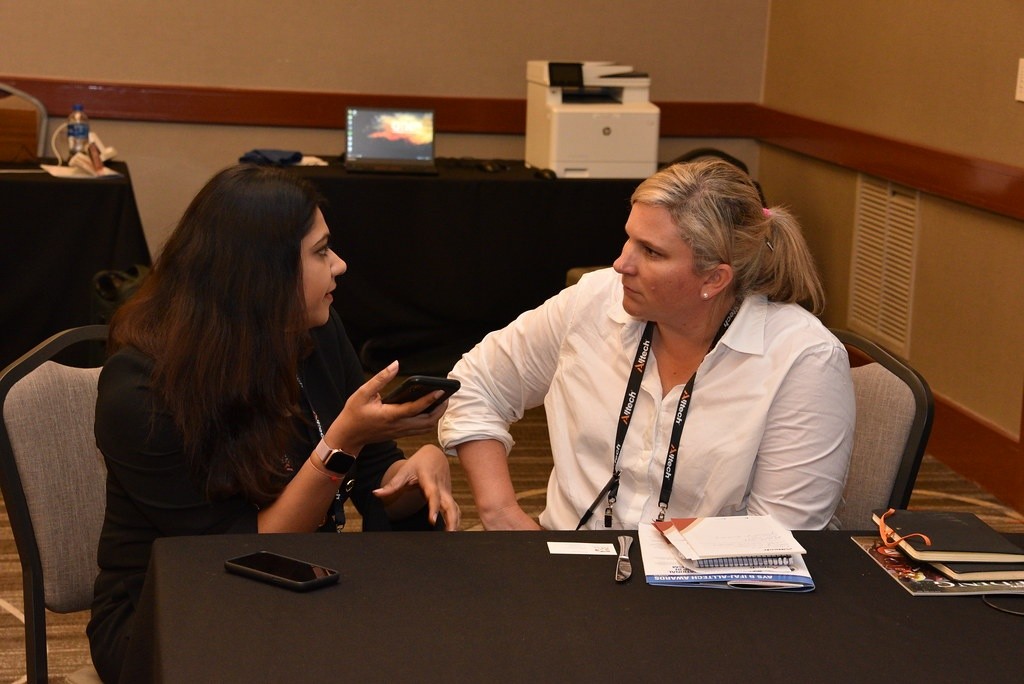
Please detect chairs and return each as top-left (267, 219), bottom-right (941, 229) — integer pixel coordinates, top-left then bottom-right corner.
top-left (0, 324), bottom-right (108, 684)
top-left (817, 323), bottom-right (932, 530)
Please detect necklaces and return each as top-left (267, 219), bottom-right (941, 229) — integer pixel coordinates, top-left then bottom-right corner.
top-left (282, 375), bottom-right (340, 502)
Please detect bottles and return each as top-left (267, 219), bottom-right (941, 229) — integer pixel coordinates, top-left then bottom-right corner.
top-left (67, 104), bottom-right (89, 162)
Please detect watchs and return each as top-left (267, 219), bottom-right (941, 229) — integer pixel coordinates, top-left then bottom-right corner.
top-left (317, 439), bottom-right (356, 475)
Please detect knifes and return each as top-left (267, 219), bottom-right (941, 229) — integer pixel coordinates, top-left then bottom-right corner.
top-left (616, 535), bottom-right (633, 583)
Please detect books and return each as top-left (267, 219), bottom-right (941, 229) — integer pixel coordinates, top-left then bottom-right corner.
top-left (851, 509), bottom-right (1024, 596)
top-left (638, 515), bottom-right (815, 592)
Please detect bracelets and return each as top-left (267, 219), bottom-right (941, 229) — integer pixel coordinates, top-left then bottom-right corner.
top-left (308, 457), bottom-right (345, 480)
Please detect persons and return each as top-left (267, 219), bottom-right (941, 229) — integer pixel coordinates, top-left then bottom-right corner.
top-left (436, 156), bottom-right (855, 529)
top-left (85, 169), bottom-right (462, 684)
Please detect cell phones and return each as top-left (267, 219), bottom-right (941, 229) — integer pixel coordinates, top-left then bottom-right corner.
top-left (381, 375), bottom-right (461, 413)
top-left (223, 550), bottom-right (340, 591)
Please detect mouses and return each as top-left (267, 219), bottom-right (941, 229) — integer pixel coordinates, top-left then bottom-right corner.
top-left (481, 161), bottom-right (506, 172)
top-left (532, 169), bottom-right (558, 180)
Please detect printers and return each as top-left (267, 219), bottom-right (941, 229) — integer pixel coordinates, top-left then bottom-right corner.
top-left (524, 61), bottom-right (661, 178)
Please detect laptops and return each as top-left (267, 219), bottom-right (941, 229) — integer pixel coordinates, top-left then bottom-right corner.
top-left (344, 105), bottom-right (440, 174)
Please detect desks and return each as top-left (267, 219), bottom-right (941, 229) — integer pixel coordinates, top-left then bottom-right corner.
top-left (0, 158), bottom-right (152, 371)
top-left (147, 527), bottom-right (1023, 684)
top-left (251, 157), bottom-right (652, 377)
